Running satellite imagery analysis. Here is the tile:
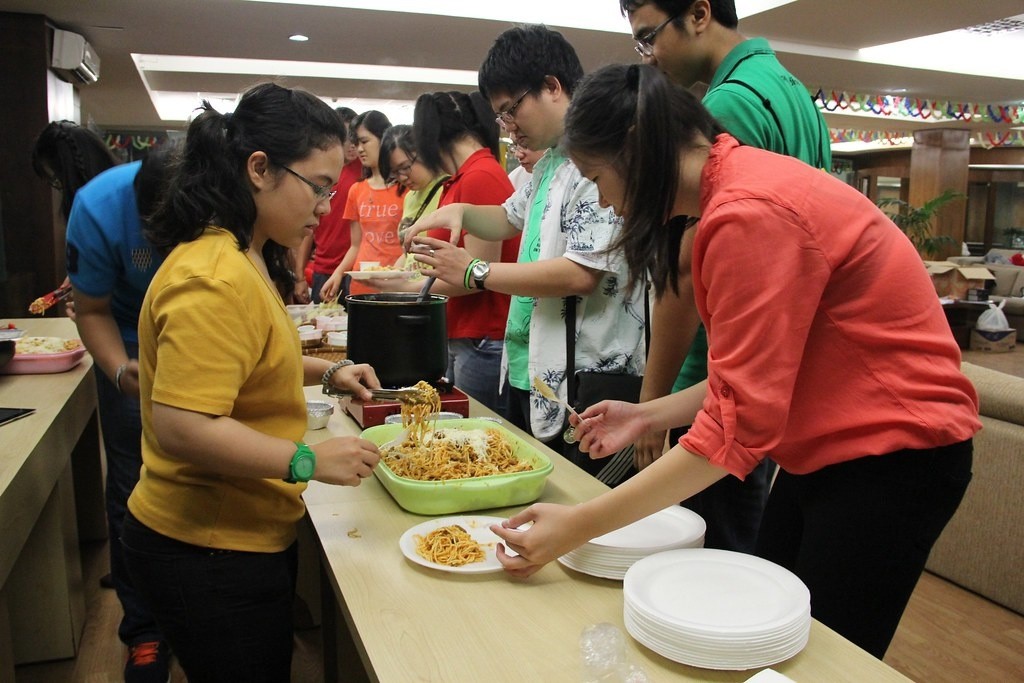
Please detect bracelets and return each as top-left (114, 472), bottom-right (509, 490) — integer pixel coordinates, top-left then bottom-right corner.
top-left (296, 276), bottom-right (306, 283)
top-left (322, 359), bottom-right (354, 395)
top-left (116, 364), bottom-right (126, 393)
top-left (464, 258), bottom-right (480, 290)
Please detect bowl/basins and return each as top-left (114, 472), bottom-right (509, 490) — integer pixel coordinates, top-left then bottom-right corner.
top-left (306, 401), bottom-right (334, 429)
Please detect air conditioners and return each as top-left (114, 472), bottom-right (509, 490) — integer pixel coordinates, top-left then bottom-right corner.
top-left (50, 27), bottom-right (100, 85)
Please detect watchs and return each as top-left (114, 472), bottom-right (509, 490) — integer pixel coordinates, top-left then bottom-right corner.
top-left (473, 260), bottom-right (490, 290)
top-left (282, 442), bottom-right (315, 484)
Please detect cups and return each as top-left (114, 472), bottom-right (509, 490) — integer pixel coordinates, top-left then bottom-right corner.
top-left (360, 261), bottom-right (380, 271)
top-left (298, 315), bottom-right (348, 346)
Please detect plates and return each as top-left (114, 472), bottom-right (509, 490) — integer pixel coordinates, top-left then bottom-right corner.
top-left (345, 272), bottom-right (417, 279)
top-left (1, 332), bottom-right (88, 374)
top-left (400, 516), bottom-right (531, 573)
top-left (558, 504), bottom-right (813, 672)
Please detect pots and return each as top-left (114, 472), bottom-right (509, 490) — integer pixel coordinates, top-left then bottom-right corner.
top-left (345, 291), bottom-right (449, 383)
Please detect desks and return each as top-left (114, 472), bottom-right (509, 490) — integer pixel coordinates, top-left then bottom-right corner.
top-left (0, 298), bottom-right (917, 683)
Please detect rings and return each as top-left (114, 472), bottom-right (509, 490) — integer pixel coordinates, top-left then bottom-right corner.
top-left (430, 249), bottom-right (434, 257)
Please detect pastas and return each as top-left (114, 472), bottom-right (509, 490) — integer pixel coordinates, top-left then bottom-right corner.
top-left (363, 265), bottom-right (408, 272)
top-left (379, 381), bottom-right (535, 481)
top-left (414, 523), bottom-right (486, 568)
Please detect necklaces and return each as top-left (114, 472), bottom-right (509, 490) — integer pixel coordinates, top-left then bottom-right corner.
top-left (368, 184), bottom-right (376, 203)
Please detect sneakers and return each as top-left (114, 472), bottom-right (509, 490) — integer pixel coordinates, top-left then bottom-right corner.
top-left (123, 638), bottom-right (171, 683)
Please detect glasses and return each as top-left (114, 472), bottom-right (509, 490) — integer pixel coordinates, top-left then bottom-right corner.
top-left (507, 141), bottom-right (527, 154)
top-left (634, 12), bottom-right (678, 58)
top-left (47, 178), bottom-right (62, 190)
top-left (387, 149), bottom-right (419, 177)
top-left (267, 156), bottom-right (337, 201)
top-left (495, 85), bottom-right (536, 128)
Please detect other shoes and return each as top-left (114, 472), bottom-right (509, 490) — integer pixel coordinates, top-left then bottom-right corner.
top-left (98, 573), bottom-right (115, 589)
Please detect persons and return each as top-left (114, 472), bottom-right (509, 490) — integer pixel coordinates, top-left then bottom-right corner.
top-left (404, 25), bottom-right (657, 489)
top-left (65, 139), bottom-right (179, 683)
top-left (293, 107), bottom-right (364, 312)
top-left (120, 82), bottom-right (381, 683)
top-left (354, 92), bottom-right (522, 413)
top-left (32, 120), bottom-right (124, 590)
top-left (502, 127), bottom-right (549, 193)
top-left (491, 63), bottom-right (983, 661)
top-left (320, 110), bottom-right (411, 303)
top-left (620, 0), bottom-right (830, 554)
top-left (378, 125), bottom-right (454, 283)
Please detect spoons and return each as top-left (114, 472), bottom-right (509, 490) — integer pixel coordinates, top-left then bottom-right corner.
top-left (534, 377), bottom-right (584, 425)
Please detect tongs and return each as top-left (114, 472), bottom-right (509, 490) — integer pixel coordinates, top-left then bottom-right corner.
top-left (30, 287), bottom-right (70, 315)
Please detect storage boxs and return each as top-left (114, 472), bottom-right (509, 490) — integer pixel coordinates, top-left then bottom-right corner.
top-left (970, 328), bottom-right (1016, 353)
top-left (925, 261), bottom-right (959, 297)
top-left (924, 261), bottom-right (996, 300)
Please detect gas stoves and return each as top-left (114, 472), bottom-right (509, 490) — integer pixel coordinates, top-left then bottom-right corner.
top-left (336, 379), bottom-right (469, 430)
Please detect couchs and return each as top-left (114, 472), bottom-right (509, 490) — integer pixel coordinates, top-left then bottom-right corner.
top-left (923, 249), bottom-right (1024, 616)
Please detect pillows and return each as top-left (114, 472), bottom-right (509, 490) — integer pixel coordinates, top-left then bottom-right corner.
top-left (973, 263), bottom-right (1018, 296)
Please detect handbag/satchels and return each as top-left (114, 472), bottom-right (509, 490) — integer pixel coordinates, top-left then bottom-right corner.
top-left (976, 298), bottom-right (1009, 332)
